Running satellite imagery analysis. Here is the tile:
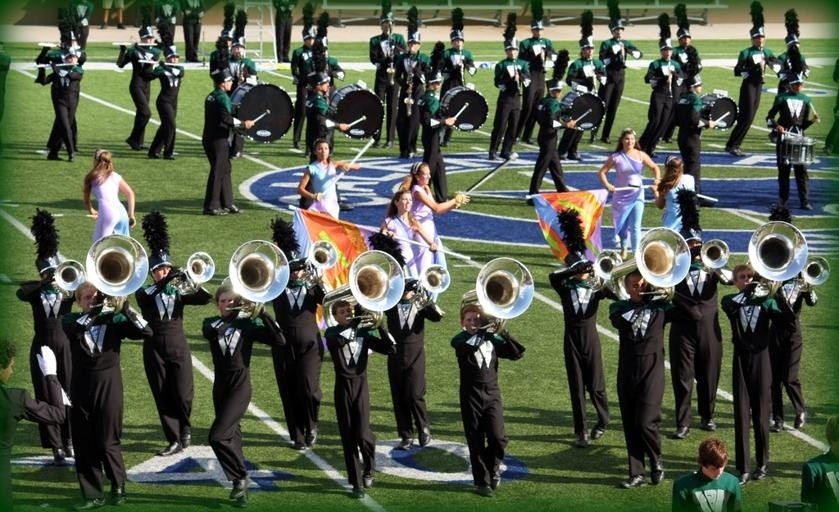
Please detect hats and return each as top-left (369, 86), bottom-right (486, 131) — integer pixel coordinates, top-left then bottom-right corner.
top-left (786, 70), bottom-right (805, 86)
top-left (308, 72), bottom-right (331, 88)
top-left (679, 225), bottom-right (704, 246)
top-left (562, 249), bottom-right (587, 269)
top-left (283, 248), bottom-right (304, 265)
top-left (146, 251), bottom-right (175, 273)
top-left (211, 67), bottom-right (236, 87)
top-left (543, 76), bottom-right (564, 93)
top-left (683, 72), bottom-right (704, 90)
top-left (43, 25), bottom-right (807, 67)
top-left (423, 69), bottom-right (446, 86)
top-left (35, 253), bottom-right (60, 277)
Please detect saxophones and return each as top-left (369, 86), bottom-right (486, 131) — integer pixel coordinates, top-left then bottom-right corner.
top-left (457, 46), bottom-right (465, 81)
top-left (403, 54), bottom-right (415, 117)
top-left (386, 22), bottom-right (396, 85)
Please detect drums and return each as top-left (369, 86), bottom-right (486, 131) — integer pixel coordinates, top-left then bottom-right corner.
top-left (233, 81), bottom-right (294, 143)
top-left (700, 92), bottom-right (739, 131)
top-left (330, 80), bottom-right (384, 140)
top-left (780, 134), bottom-right (817, 166)
top-left (560, 90), bottom-right (606, 131)
top-left (438, 86), bottom-right (489, 133)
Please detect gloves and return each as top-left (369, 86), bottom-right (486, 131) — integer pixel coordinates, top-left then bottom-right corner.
top-left (690, 247), bottom-right (703, 259)
top-left (642, 287), bottom-right (654, 306)
top-left (88, 291), bottom-right (105, 317)
top-left (37, 275), bottom-right (55, 290)
top-left (217, 304), bottom-right (241, 334)
top-left (476, 318), bottom-right (489, 343)
top-left (157, 269), bottom-right (181, 290)
top-left (562, 260), bottom-right (592, 276)
top-left (347, 304), bottom-right (366, 329)
top-left (37, 345), bottom-right (57, 377)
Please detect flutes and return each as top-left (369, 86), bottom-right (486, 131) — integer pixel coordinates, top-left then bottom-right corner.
top-left (32, 42), bottom-right (185, 67)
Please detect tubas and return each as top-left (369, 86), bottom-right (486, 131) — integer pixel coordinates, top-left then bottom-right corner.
top-left (176, 251), bottom-right (215, 296)
top-left (322, 250), bottom-right (405, 330)
top-left (413, 264), bottom-right (451, 309)
top-left (52, 260), bottom-right (86, 299)
top-left (793, 256), bottom-right (830, 292)
top-left (221, 239), bottom-right (291, 321)
top-left (610, 227), bottom-right (691, 301)
top-left (701, 239), bottom-right (736, 285)
top-left (300, 240), bottom-right (337, 289)
top-left (461, 257), bottom-right (535, 339)
top-left (746, 221), bottom-right (808, 298)
top-left (586, 249), bottom-right (624, 291)
top-left (85, 234), bottom-right (149, 314)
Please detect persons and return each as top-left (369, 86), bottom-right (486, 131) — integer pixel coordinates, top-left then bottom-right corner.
top-left (1, 0), bottom-right (839, 512)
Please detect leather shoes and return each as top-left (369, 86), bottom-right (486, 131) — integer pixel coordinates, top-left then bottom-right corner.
top-left (363, 465), bottom-right (374, 489)
top-left (418, 424), bottom-right (432, 447)
top-left (700, 417), bottom-right (717, 431)
top-left (46, 129), bottom-right (839, 213)
top-left (794, 409), bottom-right (808, 429)
top-left (111, 482), bottom-right (127, 506)
top-left (72, 496), bottom-right (106, 511)
top-left (618, 473), bottom-right (647, 489)
top-left (591, 421), bottom-right (606, 439)
top-left (158, 425), bottom-right (191, 456)
top-left (398, 436), bottom-right (413, 451)
top-left (294, 423), bottom-right (317, 450)
top-left (478, 470), bottom-right (502, 496)
top-left (576, 428), bottom-right (590, 447)
top-left (352, 475), bottom-right (363, 498)
top-left (769, 419), bottom-right (784, 432)
top-left (54, 445), bottom-right (75, 468)
top-left (230, 475), bottom-right (249, 508)
top-left (739, 462), bottom-right (768, 487)
top-left (650, 455), bottom-right (665, 485)
top-left (668, 422), bottom-right (690, 441)
